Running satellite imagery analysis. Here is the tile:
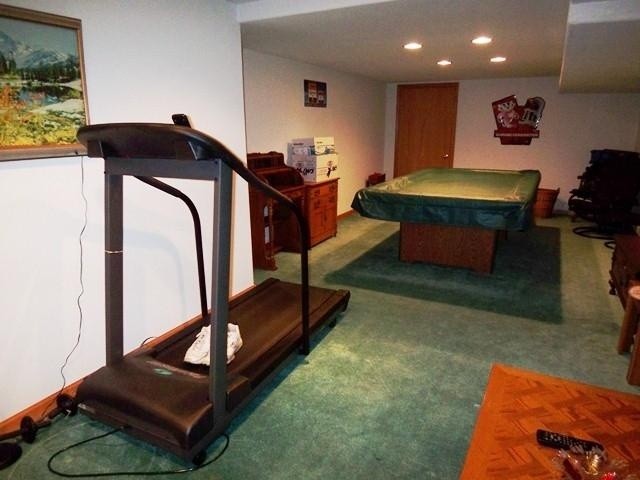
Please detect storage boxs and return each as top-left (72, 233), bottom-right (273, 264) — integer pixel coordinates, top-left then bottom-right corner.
top-left (290, 136), bottom-right (338, 183)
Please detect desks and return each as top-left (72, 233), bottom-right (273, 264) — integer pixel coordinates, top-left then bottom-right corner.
top-left (609, 234), bottom-right (640, 343)
top-left (350, 166), bottom-right (541, 274)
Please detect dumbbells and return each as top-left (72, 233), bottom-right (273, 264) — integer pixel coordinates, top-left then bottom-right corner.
top-left (21, 393), bottom-right (76, 444)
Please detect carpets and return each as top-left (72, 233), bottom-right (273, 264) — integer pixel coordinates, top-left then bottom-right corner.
top-left (460, 362), bottom-right (640, 480)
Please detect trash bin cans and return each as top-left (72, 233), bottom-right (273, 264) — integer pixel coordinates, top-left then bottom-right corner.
top-left (533, 188), bottom-right (560, 219)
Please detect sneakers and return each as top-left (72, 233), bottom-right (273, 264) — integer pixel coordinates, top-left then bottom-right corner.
top-left (203, 322), bottom-right (243, 366)
top-left (183, 323), bottom-right (211, 365)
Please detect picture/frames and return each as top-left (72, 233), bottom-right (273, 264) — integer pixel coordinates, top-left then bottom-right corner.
top-left (0, 4), bottom-right (90, 161)
top-left (304, 79), bottom-right (327, 107)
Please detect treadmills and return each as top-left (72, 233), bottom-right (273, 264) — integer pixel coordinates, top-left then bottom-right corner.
top-left (76, 113), bottom-right (350, 466)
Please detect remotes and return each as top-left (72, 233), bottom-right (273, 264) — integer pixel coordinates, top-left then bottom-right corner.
top-left (536, 429), bottom-right (604, 454)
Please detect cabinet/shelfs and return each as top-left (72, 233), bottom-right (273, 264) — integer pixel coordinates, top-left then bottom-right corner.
top-left (269, 176), bottom-right (340, 250)
